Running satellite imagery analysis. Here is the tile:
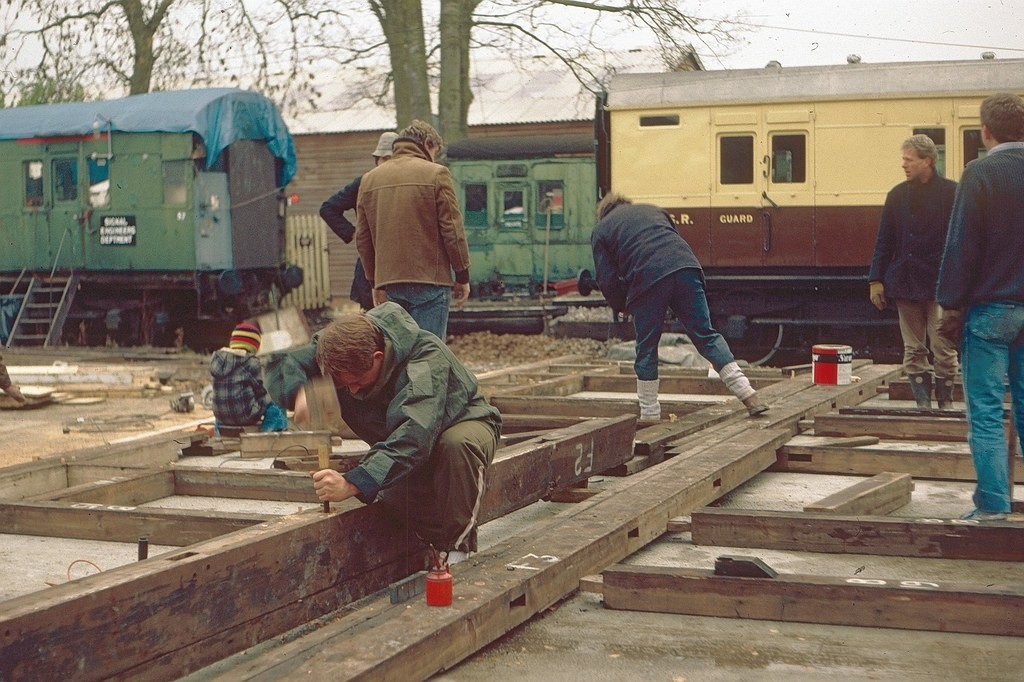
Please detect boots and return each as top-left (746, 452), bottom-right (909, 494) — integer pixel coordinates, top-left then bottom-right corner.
top-left (933, 373), bottom-right (954, 409)
top-left (908, 370), bottom-right (932, 409)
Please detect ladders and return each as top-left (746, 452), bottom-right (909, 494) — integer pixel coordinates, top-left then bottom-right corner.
top-left (6, 275), bottom-right (80, 348)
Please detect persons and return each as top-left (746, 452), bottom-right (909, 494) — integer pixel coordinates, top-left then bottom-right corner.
top-left (264, 301), bottom-right (503, 565)
top-left (867, 134), bottom-right (958, 409)
top-left (208, 323), bottom-right (288, 432)
top-left (0, 355), bottom-right (25, 402)
top-left (354, 120), bottom-right (471, 339)
top-left (934, 92), bottom-right (1024, 520)
top-left (319, 132), bottom-right (397, 311)
top-left (591, 194), bottom-right (769, 420)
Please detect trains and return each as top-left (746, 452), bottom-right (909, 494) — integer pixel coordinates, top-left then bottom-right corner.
top-left (440, 124), bottom-right (600, 299)
top-left (595, 54), bottom-right (1024, 368)
top-left (0, 86), bottom-right (295, 351)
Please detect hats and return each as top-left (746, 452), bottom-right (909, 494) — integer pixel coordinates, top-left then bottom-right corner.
top-left (229, 323), bottom-right (261, 355)
top-left (372, 132), bottom-right (400, 158)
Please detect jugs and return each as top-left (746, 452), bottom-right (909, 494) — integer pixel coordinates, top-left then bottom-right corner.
top-left (426, 544), bottom-right (453, 606)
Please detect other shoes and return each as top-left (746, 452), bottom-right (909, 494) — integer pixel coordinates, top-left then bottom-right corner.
top-left (959, 507), bottom-right (1006, 522)
top-left (744, 394), bottom-right (770, 416)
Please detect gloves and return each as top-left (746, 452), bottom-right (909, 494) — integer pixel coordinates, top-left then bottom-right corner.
top-left (935, 305), bottom-right (963, 343)
top-left (870, 280), bottom-right (887, 311)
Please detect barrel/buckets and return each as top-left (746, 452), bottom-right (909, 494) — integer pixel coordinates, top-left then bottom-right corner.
top-left (810, 344), bottom-right (853, 386)
